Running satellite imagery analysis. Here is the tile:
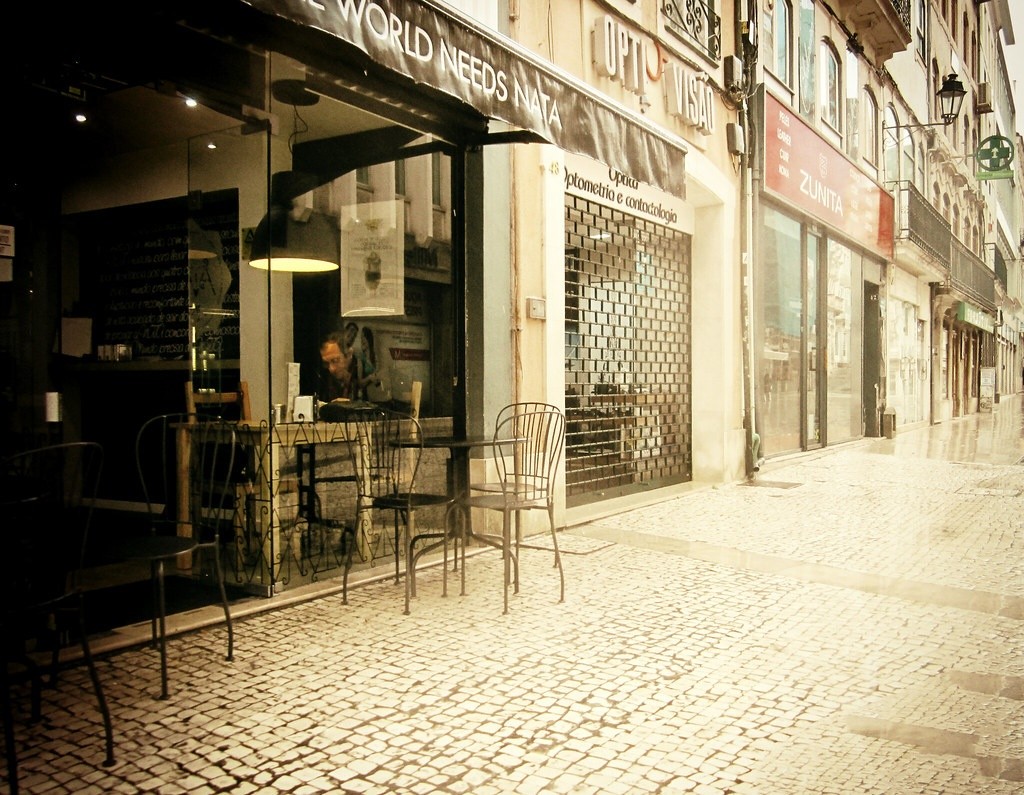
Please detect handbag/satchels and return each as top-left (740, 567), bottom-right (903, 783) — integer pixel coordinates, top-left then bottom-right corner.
top-left (367, 377), bottom-right (389, 402)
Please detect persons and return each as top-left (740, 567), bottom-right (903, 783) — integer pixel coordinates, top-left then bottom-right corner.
top-left (312, 332), bottom-right (382, 408)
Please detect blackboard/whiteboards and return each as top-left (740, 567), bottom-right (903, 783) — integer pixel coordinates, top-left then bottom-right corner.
top-left (57, 188), bottom-right (239, 359)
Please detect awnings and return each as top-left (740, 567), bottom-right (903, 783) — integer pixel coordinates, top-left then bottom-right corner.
top-left (166, 0), bottom-right (568, 173)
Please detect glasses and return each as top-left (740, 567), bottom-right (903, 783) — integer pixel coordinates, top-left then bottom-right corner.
top-left (323, 352), bottom-right (349, 369)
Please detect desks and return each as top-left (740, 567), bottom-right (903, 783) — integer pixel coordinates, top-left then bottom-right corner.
top-left (294, 417), bottom-right (455, 550)
top-left (388, 434), bottom-right (531, 598)
top-left (170, 417), bottom-right (380, 583)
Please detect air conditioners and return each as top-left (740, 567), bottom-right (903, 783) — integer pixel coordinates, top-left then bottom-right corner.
top-left (977, 82), bottom-right (995, 113)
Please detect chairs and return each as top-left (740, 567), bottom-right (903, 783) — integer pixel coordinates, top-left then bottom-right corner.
top-left (347, 379), bottom-right (422, 560)
top-left (0, 401), bottom-right (567, 795)
top-left (182, 380), bottom-right (303, 582)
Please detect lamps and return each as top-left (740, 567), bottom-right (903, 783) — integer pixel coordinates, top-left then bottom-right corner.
top-left (883, 72), bottom-right (969, 129)
top-left (248, 77), bottom-right (341, 272)
